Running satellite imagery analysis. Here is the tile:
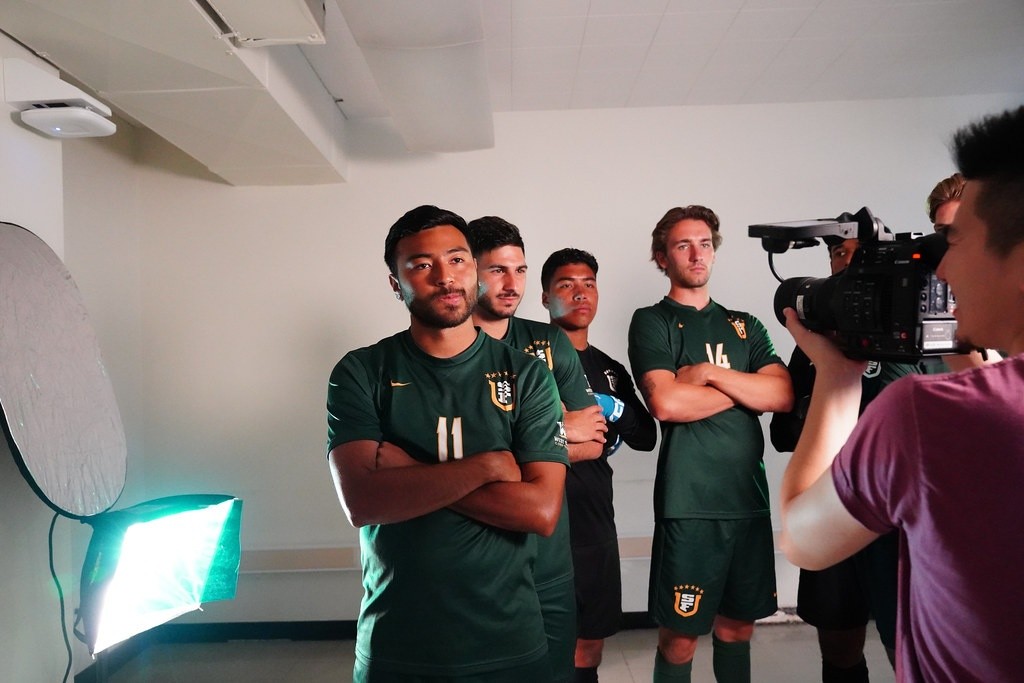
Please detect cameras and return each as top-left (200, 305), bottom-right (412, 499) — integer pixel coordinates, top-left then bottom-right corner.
top-left (749, 206), bottom-right (990, 363)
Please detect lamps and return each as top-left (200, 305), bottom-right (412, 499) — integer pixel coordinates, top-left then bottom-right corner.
top-left (76, 492), bottom-right (236, 658)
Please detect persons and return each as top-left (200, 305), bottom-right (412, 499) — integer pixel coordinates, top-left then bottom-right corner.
top-left (772, 102), bottom-right (1024, 683)
top-left (927, 173), bottom-right (966, 230)
top-left (626, 203), bottom-right (793, 683)
top-left (468, 216), bottom-right (608, 682)
top-left (540, 247), bottom-right (657, 682)
top-left (324, 203), bottom-right (571, 681)
top-left (769, 224), bottom-right (951, 683)
